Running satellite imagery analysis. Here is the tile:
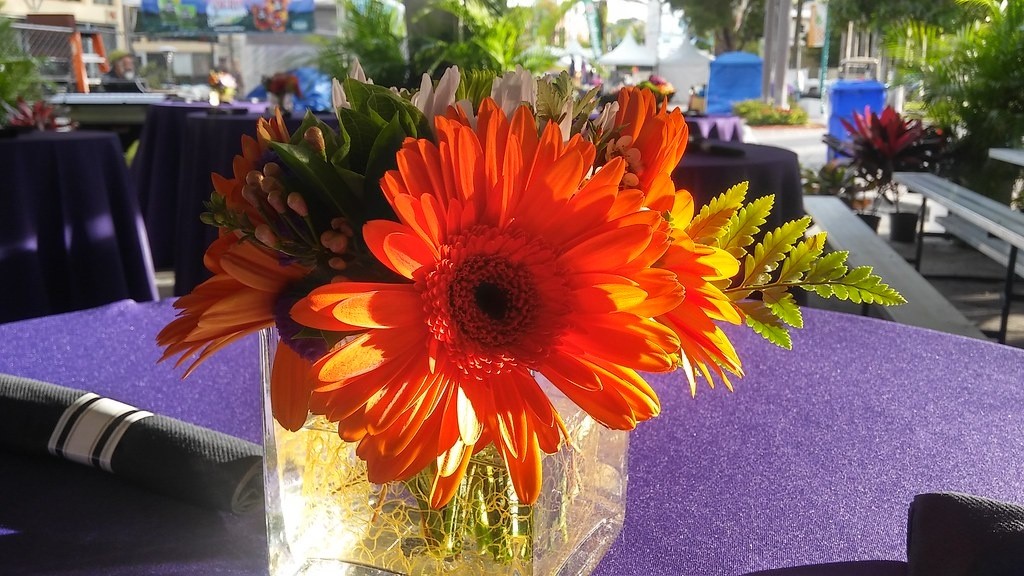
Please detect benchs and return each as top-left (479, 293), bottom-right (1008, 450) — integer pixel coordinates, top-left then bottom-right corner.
top-left (802, 195), bottom-right (990, 342)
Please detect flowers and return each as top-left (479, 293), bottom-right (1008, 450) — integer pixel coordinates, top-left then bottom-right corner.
top-left (157, 61), bottom-right (908, 575)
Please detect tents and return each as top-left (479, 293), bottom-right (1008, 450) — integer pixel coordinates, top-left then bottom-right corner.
top-left (596, 28), bottom-right (716, 104)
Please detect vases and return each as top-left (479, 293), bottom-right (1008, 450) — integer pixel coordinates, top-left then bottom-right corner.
top-left (258, 327), bottom-right (630, 576)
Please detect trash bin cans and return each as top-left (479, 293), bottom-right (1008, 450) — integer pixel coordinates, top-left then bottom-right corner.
top-left (825, 78), bottom-right (886, 162)
top-left (704, 52), bottom-right (765, 116)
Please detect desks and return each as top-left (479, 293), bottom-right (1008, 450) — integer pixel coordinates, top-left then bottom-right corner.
top-left (891, 171), bottom-right (1024, 343)
top-left (0, 101), bottom-right (343, 324)
top-left (586, 113), bottom-right (814, 308)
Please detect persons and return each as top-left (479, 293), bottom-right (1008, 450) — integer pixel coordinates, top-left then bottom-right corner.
top-left (97, 48), bottom-right (149, 93)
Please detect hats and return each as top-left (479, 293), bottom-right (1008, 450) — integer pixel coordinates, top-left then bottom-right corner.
top-left (109, 47), bottom-right (134, 63)
